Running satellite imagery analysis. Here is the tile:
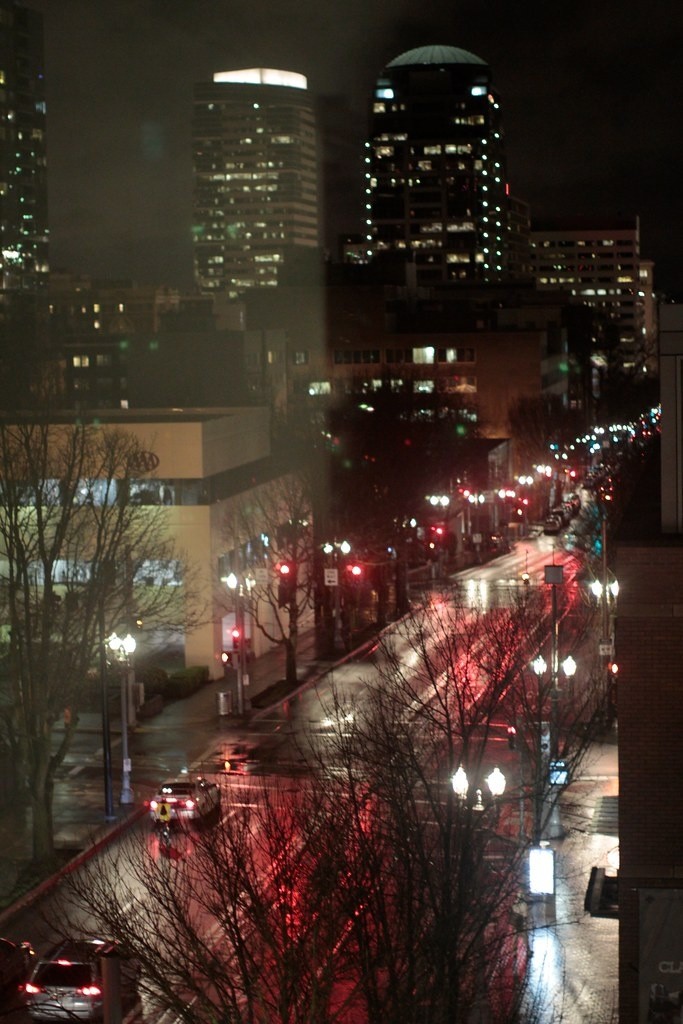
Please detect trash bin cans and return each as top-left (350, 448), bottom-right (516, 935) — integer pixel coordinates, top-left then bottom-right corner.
top-left (214, 687), bottom-right (234, 716)
top-left (517, 522), bottom-right (525, 537)
top-left (428, 563), bottom-right (439, 580)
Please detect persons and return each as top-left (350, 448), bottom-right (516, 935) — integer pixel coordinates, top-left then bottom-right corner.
top-left (65, 706), bottom-right (72, 735)
top-left (155, 796), bottom-right (172, 848)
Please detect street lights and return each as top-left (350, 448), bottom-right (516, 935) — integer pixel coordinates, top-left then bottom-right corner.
top-left (530, 654), bottom-right (548, 848)
top-left (226, 572), bottom-right (255, 717)
top-left (561, 655), bottom-right (578, 761)
top-left (108, 630), bottom-right (136, 805)
top-left (445, 764), bottom-right (505, 1024)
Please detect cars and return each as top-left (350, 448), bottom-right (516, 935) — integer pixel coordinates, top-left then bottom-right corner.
top-left (21, 937), bottom-right (141, 1024)
top-left (0, 935), bottom-right (38, 993)
top-left (544, 493), bottom-right (581, 535)
top-left (150, 776), bottom-right (222, 828)
